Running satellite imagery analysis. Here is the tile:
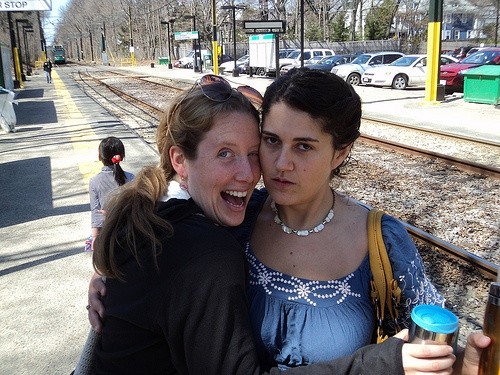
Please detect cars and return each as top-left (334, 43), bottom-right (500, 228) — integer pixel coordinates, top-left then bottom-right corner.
top-left (301, 52), bottom-right (356, 74)
top-left (219, 49), bottom-right (294, 75)
top-left (176, 49), bottom-right (234, 70)
top-left (438, 47), bottom-right (500, 91)
top-left (330, 51), bottom-right (406, 88)
top-left (362, 55), bottom-right (460, 91)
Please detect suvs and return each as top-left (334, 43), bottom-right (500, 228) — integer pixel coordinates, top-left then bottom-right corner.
top-left (279, 48), bottom-right (338, 77)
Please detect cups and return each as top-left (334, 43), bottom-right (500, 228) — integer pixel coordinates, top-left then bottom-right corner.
top-left (477, 282), bottom-right (500, 375)
top-left (406, 304), bottom-right (460, 370)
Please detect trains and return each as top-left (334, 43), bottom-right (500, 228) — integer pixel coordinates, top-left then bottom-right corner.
top-left (53, 44), bottom-right (65, 65)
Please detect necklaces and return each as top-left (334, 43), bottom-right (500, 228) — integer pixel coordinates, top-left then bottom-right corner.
top-left (271, 186), bottom-right (335, 236)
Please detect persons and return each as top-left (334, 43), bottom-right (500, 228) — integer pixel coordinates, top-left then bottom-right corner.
top-left (43, 57), bottom-right (52, 84)
top-left (85, 136), bottom-right (136, 255)
top-left (71, 67), bottom-right (488, 375)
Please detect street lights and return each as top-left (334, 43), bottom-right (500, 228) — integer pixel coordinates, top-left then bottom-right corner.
top-left (184, 14), bottom-right (203, 56)
top-left (221, 5), bottom-right (247, 75)
top-left (7, 11), bottom-right (33, 81)
top-left (159, 16), bottom-right (176, 68)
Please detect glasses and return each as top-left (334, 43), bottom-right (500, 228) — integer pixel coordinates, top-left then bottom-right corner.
top-left (172, 74), bottom-right (265, 115)
